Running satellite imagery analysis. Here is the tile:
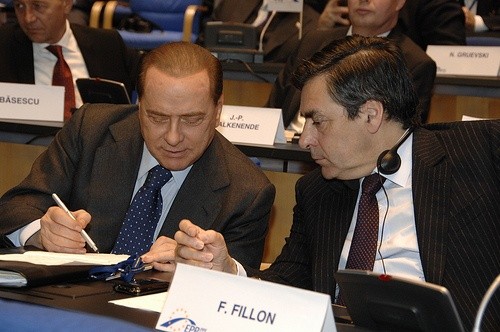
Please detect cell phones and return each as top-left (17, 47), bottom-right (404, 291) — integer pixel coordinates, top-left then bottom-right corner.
top-left (115, 278), bottom-right (170, 297)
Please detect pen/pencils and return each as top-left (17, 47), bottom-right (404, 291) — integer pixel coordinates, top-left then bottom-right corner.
top-left (52, 193), bottom-right (100, 254)
top-left (469, 0), bottom-right (477, 11)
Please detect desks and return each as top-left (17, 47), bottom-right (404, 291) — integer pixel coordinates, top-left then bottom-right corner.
top-left (0, 57), bottom-right (500, 332)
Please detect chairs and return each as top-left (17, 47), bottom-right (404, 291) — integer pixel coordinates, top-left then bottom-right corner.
top-left (89, 0), bottom-right (210, 51)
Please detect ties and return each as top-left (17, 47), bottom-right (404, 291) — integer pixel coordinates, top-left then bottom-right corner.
top-left (345, 174), bottom-right (387, 271)
top-left (112, 165), bottom-right (173, 255)
top-left (46, 45), bottom-right (76, 121)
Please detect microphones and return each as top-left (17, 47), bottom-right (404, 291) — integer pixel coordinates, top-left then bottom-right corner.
top-left (377, 122), bottom-right (416, 174)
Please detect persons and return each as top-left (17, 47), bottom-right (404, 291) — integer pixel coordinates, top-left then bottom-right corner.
top-left (0, 41), bottom-right (276, 272)
top-left (0, 0), bottom-right (136, 123)
top-left (264, 0), bottom-right (437, 125)
top-left (174, 35), bottom-right (500, 332)
top-left (194, 0), bottom-right (320, 62)
top-left (400, 0), bottom-right (500, 52)
